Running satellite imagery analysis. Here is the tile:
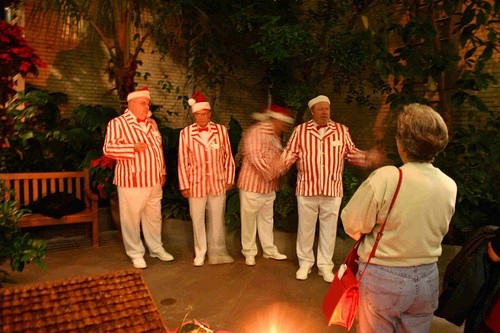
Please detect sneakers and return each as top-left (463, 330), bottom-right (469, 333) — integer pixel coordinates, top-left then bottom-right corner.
top-left (318, 270), bottom-right (335, 282)
top-left (295, 266), bottom-right (311, 279)
top-left (193, 256), bottom-right (204, 266)
top-left (263, 251), bottom-right (288, 259)
top-left (209, 254), bottom-right (235, 264)
top-left (245, 256), bottom-right (256, 265)
top-left (132, 257), bottom-right (147, 268)
top-left (150, 250), bottom-right (174, 261)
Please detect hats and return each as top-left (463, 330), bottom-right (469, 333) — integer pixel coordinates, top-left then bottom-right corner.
top-left (266, 102), bottom-right (295, 124)
top-left (308, 95), bottom-right (331, 110)
top-left (187, 90), bottom-right (211, 113)
top-left (127, 87), bottom-right (151, 101)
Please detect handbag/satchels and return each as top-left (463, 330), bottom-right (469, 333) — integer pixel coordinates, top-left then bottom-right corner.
top-left (322, 264), bottom-right (361, 331)
top-left (344, 238), bottom-right (360, 274)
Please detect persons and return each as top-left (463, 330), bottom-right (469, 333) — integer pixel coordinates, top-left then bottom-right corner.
top-left (433, 225), bottom-right (500, 333)
top-left (103, 87), bottom-right (174, 269)
top-left (177, 91), bottom-right (236, 267)
top-left (236, 104), bottom-right (295, 266)
top-left (340, 103), bottom-right (458, 333)
top-left (276, 94), bottom-right (369, 283)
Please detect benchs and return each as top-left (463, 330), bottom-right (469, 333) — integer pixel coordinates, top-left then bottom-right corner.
top-left (0, 168), bottom-right (100, 246)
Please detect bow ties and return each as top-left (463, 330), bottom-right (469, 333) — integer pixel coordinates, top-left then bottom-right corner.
top-left (316, 123), bottom-right (327, 130)
top-left (137, 117), bottom-right (147, 123)
top-left (198, 127), bottom-right (208, 132)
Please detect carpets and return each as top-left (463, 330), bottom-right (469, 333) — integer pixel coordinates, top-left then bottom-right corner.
top-left (0, 267), bottom-right (170, 333)
